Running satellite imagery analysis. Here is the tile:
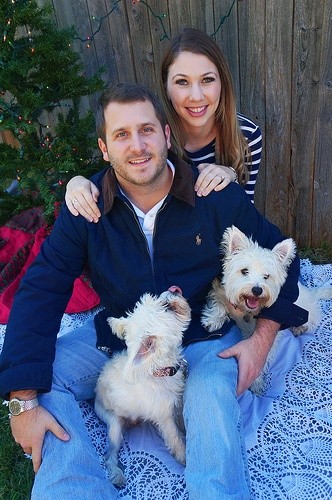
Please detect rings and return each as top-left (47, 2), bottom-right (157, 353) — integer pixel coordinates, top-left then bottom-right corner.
top-left (220, 176), bottom-right (224, 180)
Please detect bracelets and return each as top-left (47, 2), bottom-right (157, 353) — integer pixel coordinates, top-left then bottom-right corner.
top-left (229, 166), bottom-right (237, 181)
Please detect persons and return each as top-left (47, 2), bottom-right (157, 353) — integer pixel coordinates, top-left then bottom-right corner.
top-left (64, 30), bottom-right (264, 224)
top-left (0, 81), bottom-right (308, 500)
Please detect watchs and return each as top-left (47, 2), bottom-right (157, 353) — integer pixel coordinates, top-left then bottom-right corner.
top-left (9, 396), bottom-right (39, 416)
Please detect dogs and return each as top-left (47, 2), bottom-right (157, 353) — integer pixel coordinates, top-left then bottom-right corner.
top-left (92, 285), bottom-right (192, 491)
top-left (202, 224), bottom-right (332, 338)
